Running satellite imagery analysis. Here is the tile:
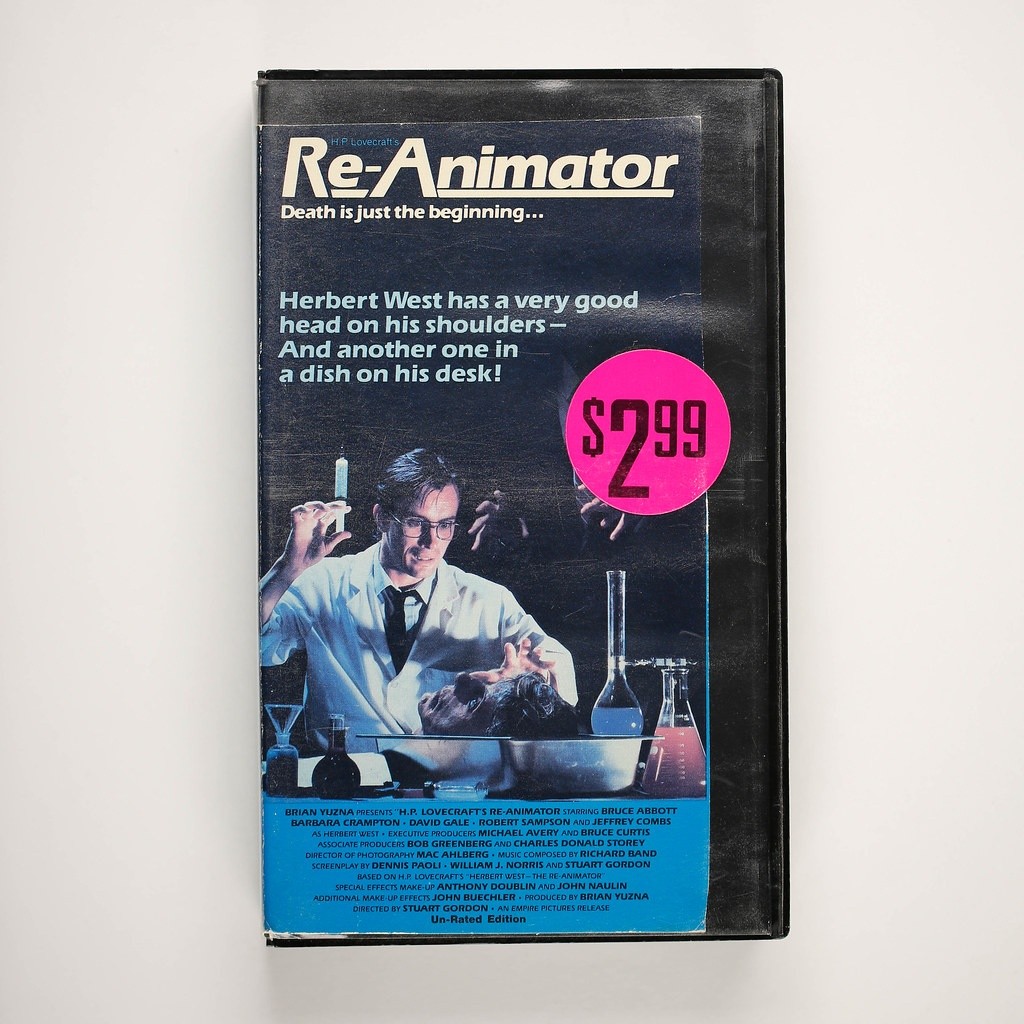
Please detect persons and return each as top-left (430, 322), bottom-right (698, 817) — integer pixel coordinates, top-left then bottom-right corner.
top-left (258, 443), bottom-right (582, 785)
top-left (470, 486), bottom-right (645, 555)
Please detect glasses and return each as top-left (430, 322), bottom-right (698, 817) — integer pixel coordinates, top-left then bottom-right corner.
top-left (389, 511), bottom-right (462, 540)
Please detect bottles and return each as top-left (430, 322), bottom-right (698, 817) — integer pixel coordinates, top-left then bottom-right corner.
top-left (313, 714), bottom-right (361, 798)
top-left (266, 733), bottom-right (298, 794)
top-left (593, 571), bottom-right (643, 736)
top-left (642, 669), bottom-right (708, 798)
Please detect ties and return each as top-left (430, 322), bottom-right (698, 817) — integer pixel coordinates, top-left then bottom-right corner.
top-left (381, 586), bottom-right (416, 676)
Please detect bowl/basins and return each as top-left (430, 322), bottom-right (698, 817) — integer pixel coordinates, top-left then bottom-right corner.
top-left (358, 735), bottom-right (513, 795)
top-left (499, 735), bottom-right (667, 793)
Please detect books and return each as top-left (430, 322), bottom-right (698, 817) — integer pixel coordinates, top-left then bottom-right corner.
top-left (252, 64), bottom-right (791, 946)
